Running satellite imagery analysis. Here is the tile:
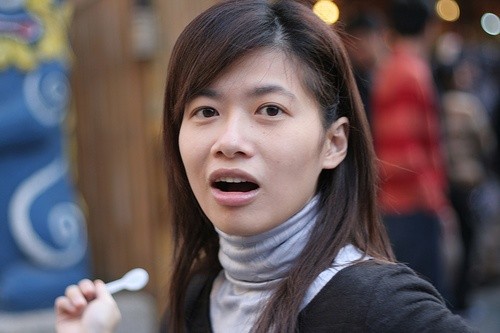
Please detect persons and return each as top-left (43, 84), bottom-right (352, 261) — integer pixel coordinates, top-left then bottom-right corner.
top-left (338, 2), bottom-right (500, 323)
top-left (55, 1), bottom-right (473, 333)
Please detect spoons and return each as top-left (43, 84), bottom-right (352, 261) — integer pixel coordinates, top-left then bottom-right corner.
top-left (105, 268), bottom-right (150, 294)
